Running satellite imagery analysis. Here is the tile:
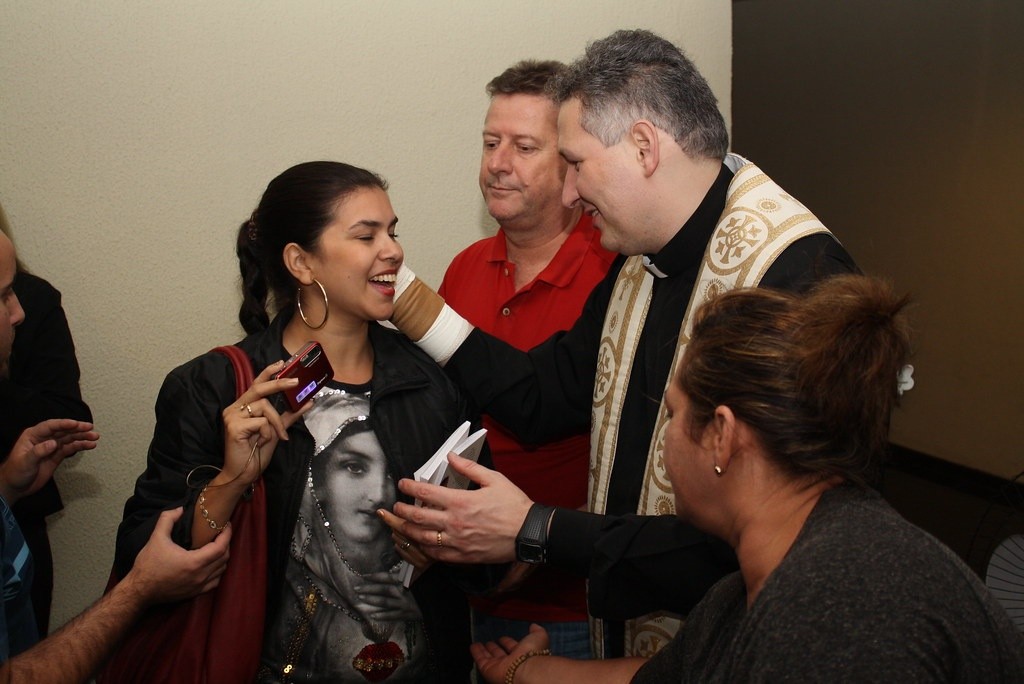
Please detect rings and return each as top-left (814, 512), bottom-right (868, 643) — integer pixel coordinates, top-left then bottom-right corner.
top-left (437, 531), bottom-right (443, 548)
top-left (244, 402), bottom-right (254, 417)
top-left (400, 540), bottom-right (410, 549)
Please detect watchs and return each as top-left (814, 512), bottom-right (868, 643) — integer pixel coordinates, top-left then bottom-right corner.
top-left (513, 502), bottom-right (555, 564)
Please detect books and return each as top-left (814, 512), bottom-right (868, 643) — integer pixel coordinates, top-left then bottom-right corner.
top-left (395, 420), bottom-right (490, 590)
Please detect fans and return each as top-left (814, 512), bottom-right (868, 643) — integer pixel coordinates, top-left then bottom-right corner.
top-left (964, 471), bottom-right (1024, 637)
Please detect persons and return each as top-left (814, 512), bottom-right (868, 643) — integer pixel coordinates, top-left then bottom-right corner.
top-left (442, 62), bottom-right (618, 684)
top-left (473, 273), bottom-right (1024, 683)
top-left (0, 207), bottom-right (94, 657)
top-left (389, 31), bottom-right (889, 652)
top-left (0, 223), bottom-right (231, 684)
top-left (94, 162), bottom-right (514, 684)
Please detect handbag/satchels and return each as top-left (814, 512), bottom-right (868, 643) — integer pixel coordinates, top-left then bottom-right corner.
top-left (78, 346), bottom-right (268, 684)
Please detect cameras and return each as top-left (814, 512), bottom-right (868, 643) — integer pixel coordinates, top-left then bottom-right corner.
top-left (272, 341), bottom-right (334, 414)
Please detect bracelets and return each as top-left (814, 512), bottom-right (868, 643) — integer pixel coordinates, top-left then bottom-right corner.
top-left (504, 649), bottom-right (552, 684)
top-left (199, 484), bottom-right (229, 534)
